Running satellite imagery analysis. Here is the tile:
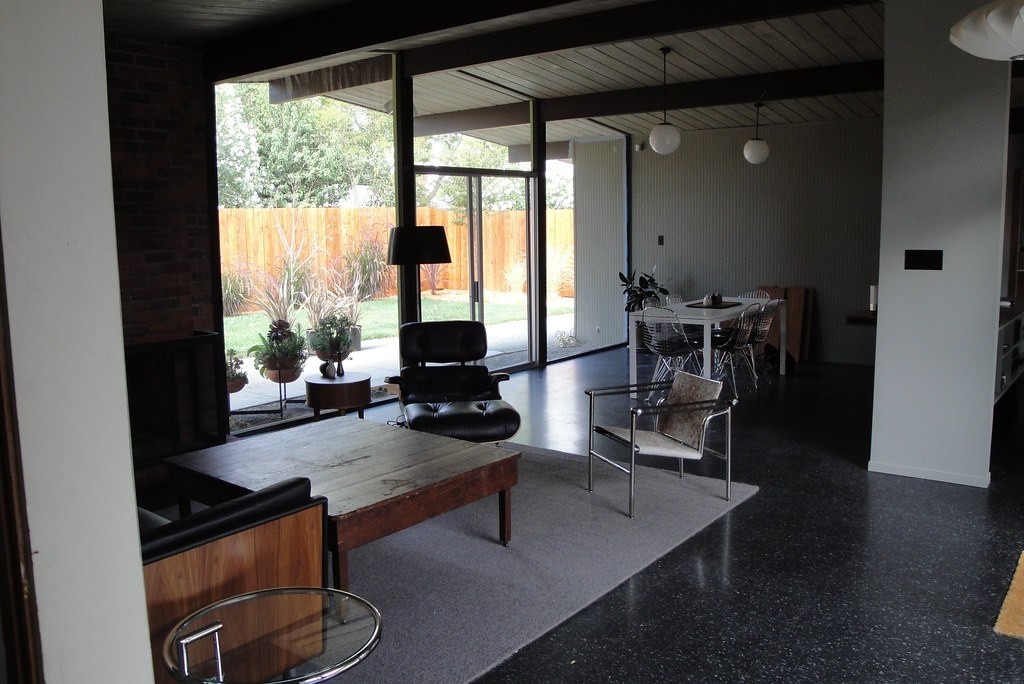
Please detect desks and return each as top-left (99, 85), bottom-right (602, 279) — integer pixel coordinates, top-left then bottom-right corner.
top-left (628, 297), bottom-right (787, 398)
top-left (171, 413), bottom-right (522, 626)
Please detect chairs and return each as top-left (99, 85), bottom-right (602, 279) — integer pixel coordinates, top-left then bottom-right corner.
top-left (666, 294), bottom-right (719, 336)
top-left (718, 290), bottom-right (771, 363)
top-left (385, 321), bottom-right (521, 446)
top-left (643, 298), bottom-right (700, 345)
top-left (697, 301), bottom-right (761, 398)
top-left (732, 298), bottom-right (781, 387)
top-left (641, 307), bottom-right (701, 404)
top-left (586, 370), bottom-right (735, 519)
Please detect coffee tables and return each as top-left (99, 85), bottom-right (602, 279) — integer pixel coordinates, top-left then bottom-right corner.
top-left (160, 584), bottom-right (384, 684)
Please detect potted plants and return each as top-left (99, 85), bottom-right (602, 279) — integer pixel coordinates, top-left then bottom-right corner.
top-left (299, 283), bottom-right (370, 354)
top-left (225, 348), bottom-right (249, 393)
top-left (311, 314), bottom-right (353, 362)
top-left (247, 319), bottom-right (307, 384)
top-left (619, 267), bottom-right (672, 349)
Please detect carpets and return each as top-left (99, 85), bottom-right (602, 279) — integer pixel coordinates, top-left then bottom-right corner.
top-left (290, 442), bottom-right (763, 683)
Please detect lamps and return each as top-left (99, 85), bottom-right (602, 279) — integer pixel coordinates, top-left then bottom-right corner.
top-left (744, 103), bottom-right (769, 164)
top-left (649, 47), bottom-right (681, 155)
top-left (386, 227), bottom-right (452, 366)
top-left (949, 0), bottom-right (1024, 61)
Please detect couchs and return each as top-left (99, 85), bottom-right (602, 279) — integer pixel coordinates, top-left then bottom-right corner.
top-left (138, 478), bottom-right (329, 684)
